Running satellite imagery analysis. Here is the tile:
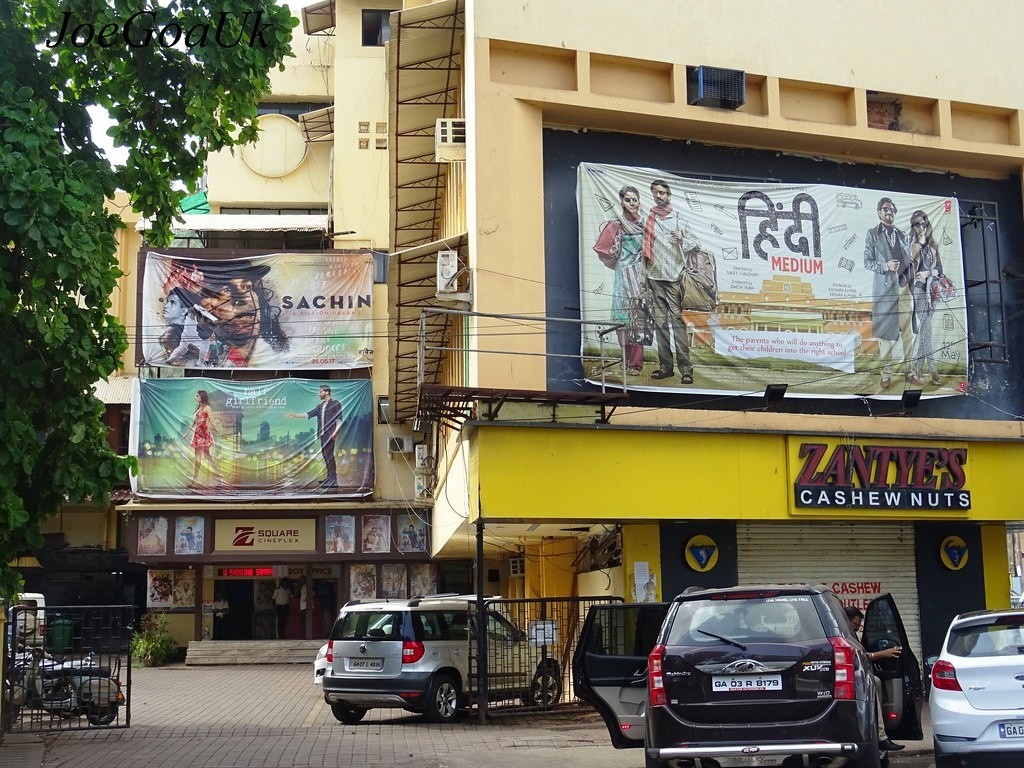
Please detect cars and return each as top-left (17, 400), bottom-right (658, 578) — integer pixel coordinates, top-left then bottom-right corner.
top-left (313, 596), bottom-right (512, 689)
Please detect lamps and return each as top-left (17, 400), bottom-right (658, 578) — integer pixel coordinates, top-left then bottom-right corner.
top-left (902, 389), bottom-right (922, 407)
top-left (762, 384), bottom-right (788, 412)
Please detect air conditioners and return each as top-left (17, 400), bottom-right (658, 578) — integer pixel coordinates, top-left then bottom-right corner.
top-left (507, 558), bottom-right (525, 577)
top-left (435, 118), bottom-right (466, 162)
top-left (387, 436), bottom-right (414, 453)
top-left (415, 445), bottom-right (427, 469)
top-left (437, 251), bottom-right (457, 294)
top-left (415, 474), bottom-right (426, 499)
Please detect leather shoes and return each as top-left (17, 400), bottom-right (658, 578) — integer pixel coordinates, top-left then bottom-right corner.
top-left (880, 739), bottom-right (906, 750)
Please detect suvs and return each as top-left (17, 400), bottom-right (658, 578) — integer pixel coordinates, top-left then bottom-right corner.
top-left (0, 592), bottom-right (46, 657)
top-left (924, 609), bottom-right (1024, 768)
top-left (572, 580), bottom-right (925, 768)
top-left (321, 593), bottom-right (563, 725)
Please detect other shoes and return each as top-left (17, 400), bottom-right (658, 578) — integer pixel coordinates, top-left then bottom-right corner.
top-left (906, 375), bottom-right (924, 385)
top-left (880, 377), bottom-right (891, 388)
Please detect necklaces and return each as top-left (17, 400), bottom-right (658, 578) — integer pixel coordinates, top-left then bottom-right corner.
top-left (920, 244), bottom-right (935, 263)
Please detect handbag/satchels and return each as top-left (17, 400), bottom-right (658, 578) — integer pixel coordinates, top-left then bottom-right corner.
top-left (627, 296), bottom-right (655, 346)
top-left (678, 247), bottom-right (719, 313)
top-left (932, 266), bottom-right (959, 303)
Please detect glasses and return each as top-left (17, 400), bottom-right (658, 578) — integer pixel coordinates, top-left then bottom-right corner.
top-left (850, 618), bottom-right (862, 629)
top-left (914, 222), bottom-right (927, 228)
top-left (882, 207), bottom-right (894, 212)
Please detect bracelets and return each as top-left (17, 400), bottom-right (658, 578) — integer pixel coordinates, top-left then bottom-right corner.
top-left (870, 652), bottom-right (875, 660)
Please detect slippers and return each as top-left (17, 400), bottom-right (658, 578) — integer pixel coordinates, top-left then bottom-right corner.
top-left (681, 374), bottom-right (693, 384)
top-left (651, 369), bottom-right (674, 378)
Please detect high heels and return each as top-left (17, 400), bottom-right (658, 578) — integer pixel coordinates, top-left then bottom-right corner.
top-left (929, 371), bottom-right (941, 385)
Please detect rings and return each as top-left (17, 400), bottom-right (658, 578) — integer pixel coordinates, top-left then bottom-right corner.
top-left (894, 647), bottom-right (897, 650)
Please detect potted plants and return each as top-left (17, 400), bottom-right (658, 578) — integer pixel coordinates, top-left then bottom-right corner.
top-left (128, 605), bottom-right (179, 667)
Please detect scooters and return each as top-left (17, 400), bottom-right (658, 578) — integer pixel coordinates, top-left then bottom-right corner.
top-left (0, 647), bottom-right (125, 727)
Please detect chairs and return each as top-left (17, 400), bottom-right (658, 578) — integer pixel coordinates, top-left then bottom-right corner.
top-left (368, 629), bottom-right (385, 637)
top-left (450, 613), bottom-right (468, 638)
top-left (967, 634), bottom-right (994, 653)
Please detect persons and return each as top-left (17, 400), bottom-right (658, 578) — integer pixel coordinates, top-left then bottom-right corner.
top-left (300, 574), bottom-right (314, 639)
top-left (180, 582), bottom-right (192, 602)
top-left (846, 606), bottom-right (905, 751)
top-left (273, 576), bottom-right (292, 638)
top-left (180, 527), bottom-right (202, 550)
top-left (371, 527), bottom-right (386, 550)
top-left (403, 524), bottom-right (425, 548)
top-left (162, 262), bottom-right (278, 364)
top-left (182, 390), bottom-right (227, 487)
top-left (865, 197), bottom-right (944, 389)
top-left (595, 181), bottom-right (699, 383)
top-left (286, 385), bottom-right (342, 487)
